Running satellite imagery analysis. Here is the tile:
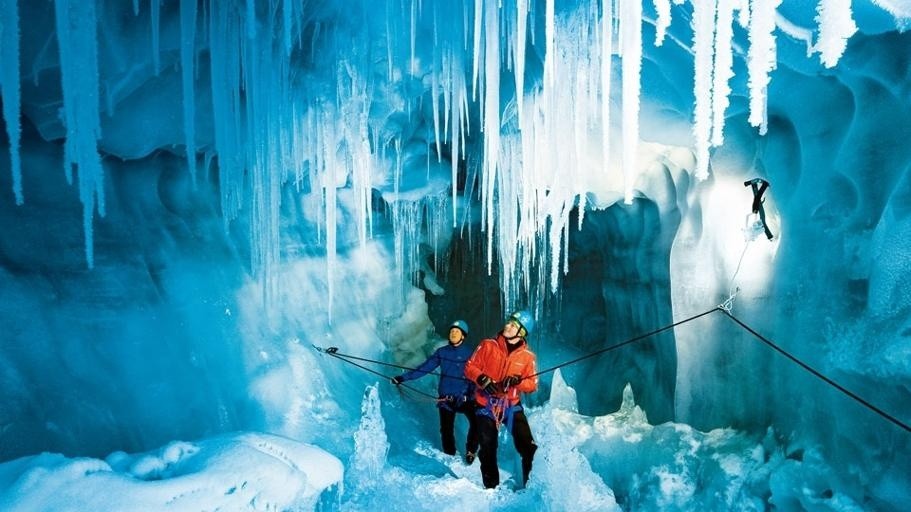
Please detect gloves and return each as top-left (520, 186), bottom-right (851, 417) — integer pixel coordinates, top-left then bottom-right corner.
top-left (392, 376), bottom-right (404, 385)
top-left (501, 374), bottom-right (522, 394)
top-left (476, 374), bottom-right (498, 397)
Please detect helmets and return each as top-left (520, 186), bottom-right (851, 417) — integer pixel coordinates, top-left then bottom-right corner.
top-left (449, 320), bottom-right (469, 336)
top-left (510, 310), bottom-right (534, 336)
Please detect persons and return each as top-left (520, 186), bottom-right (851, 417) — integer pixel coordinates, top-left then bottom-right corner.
top-left (464, 308), bottom-right (540, 491)
top-left (389, 320), bottom-right (482, 466)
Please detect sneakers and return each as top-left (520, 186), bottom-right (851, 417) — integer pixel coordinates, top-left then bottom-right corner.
top-left (466, 443), bottom-right (475, 465)
